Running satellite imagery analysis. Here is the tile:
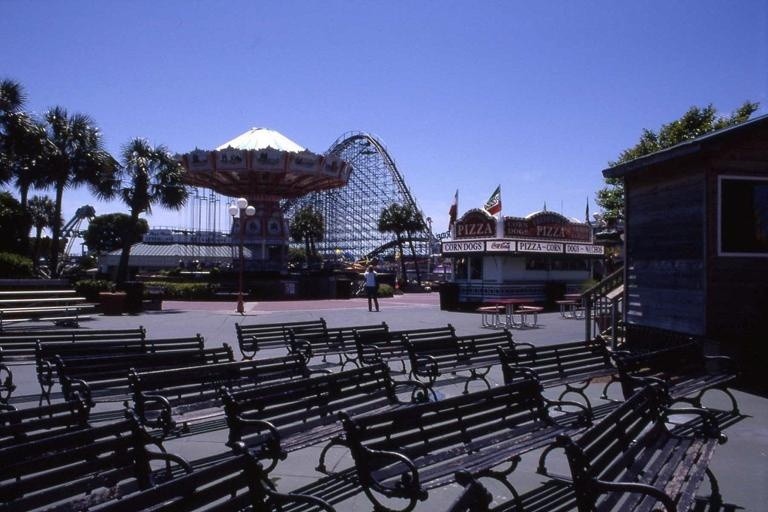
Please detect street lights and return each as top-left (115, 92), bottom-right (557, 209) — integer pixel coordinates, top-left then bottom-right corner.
top-left (229, 198), bottom-right (256, 313)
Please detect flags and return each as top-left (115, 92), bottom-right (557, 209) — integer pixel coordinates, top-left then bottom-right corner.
top-left (484, 186), bottom-right (502, 215)
top-left (449, 190), bottom-right (459, 221)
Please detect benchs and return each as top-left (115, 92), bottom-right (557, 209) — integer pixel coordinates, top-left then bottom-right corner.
top-left (2, 289), bottom-right (98, 327)
top-left (2, 317), bottom-right (747, 512)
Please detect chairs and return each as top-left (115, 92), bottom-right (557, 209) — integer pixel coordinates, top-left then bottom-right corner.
top-left (479, 297), bottom-right (543, 332)
top-left (556, 293), bottom-right (612, 319)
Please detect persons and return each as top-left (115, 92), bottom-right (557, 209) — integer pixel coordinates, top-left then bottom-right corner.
top-left (362, 265), bottom-right (380, 311)
top-left (127, 273), bottom-right (145, 318)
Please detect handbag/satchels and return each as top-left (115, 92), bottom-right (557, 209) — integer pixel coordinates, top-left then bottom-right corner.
top-left (375, 276), bottom-right (379, 289)
